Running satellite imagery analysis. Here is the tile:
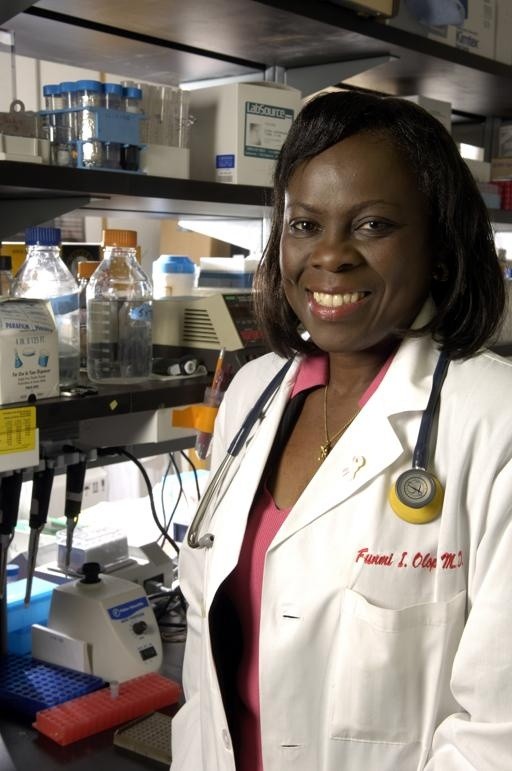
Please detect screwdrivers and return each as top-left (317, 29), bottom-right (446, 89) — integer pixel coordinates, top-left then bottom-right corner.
top-left (0, 442), bottom-right (88, 610)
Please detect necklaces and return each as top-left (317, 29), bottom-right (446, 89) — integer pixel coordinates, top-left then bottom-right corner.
top-left (317, 385), bottom-right (360, 462)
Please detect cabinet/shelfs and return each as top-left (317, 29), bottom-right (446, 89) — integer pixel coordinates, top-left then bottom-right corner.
top-left (1, 1), bottom-right (511, 770)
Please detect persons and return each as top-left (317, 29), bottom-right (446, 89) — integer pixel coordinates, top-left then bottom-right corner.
top-left (169, 91), bottom-right (512, 771)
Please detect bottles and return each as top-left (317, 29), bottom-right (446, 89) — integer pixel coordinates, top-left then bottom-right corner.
top-left (110, 679), bottom-right (120, 700)
top-left (7, 223), bottom-right (194, 390)
top-left (43, 77), bottom-right (187, 177)
